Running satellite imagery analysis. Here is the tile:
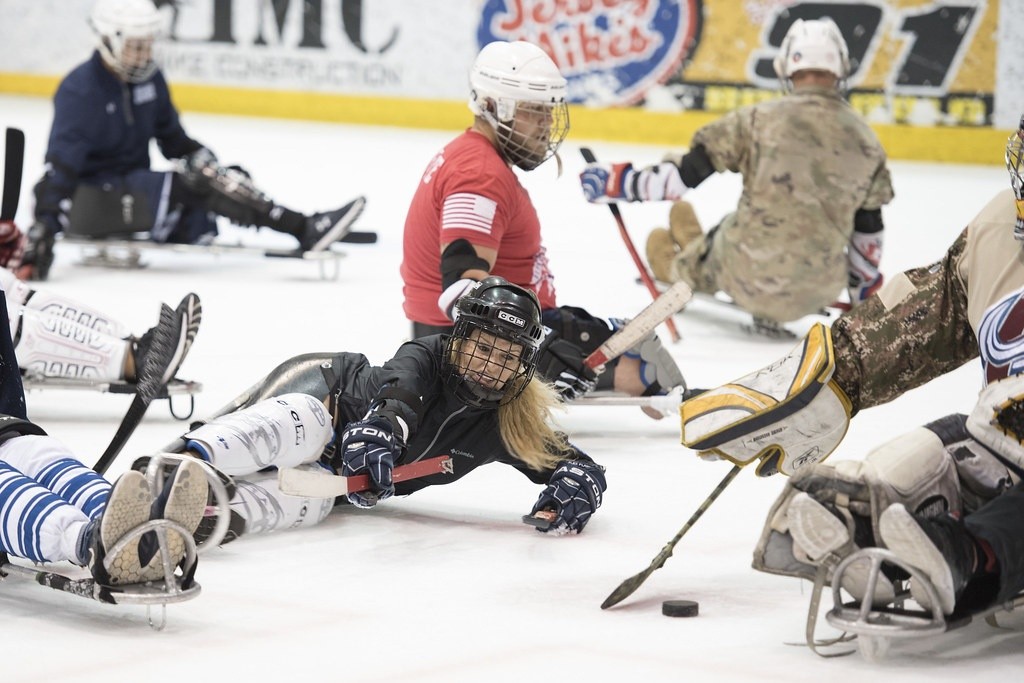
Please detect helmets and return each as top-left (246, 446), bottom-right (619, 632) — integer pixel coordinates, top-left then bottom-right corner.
top-left (88, 1), bottom-right (164, 83)
top-left (446, 281), bottom-right (544, 413)
top-left (470, 41), bottom-right (571, 171)
top-left (774, 15), bottom-right (849, 78)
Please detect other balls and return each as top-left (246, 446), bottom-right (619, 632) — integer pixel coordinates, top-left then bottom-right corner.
top-left (662, 600), bottom-right (698, 618)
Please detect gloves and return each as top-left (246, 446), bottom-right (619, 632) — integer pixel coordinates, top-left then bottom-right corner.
top-left (16, 224), bottom-right (58, 282)
top-left (583, 161), bottom-right (644, 205)
top-left (531, 461), bottom-right (607, 538)
top-left (341, 420), bottom-right (403, 509)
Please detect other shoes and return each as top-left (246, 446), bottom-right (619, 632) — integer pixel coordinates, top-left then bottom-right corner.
top-left (786, 493), bottom-right (892, 613)
top-left (881, 503), bottom-right (965, 615)
top-left (126, 292), bottom-right (203, 393)
top-left (137, 462), bottom-right (208, 585)
top-left (99, 469), bottom-right (152, 585)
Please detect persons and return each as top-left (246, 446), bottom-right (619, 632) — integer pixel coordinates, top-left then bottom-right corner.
top-left (0, 266), bottom-right (202, 386)
top-left (580, 17), bottom-right (895, 324)
top-left (0, 412), bottom-right (208, 586)
top-left (12, 0), bottom-right (367, 284)
top-left (400, 41), bottom-right (682, 398)
top-left (680, 114), bottom-right (1024, 616)
top-left (131, 276), bottom-right (607, 547)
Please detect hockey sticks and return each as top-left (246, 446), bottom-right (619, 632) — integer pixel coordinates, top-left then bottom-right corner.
top-left (581, 278), bottom-right (693, 370)
top-left (600, 465), bottom-right (745, 610)
top-left (580, 147), bottom-right (682, 344)
top-left (278, 454), bottom-right (451, 499)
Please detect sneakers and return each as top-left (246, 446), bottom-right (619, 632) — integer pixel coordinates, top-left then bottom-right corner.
top-left (302, 197), bottom-right (365, 253)
